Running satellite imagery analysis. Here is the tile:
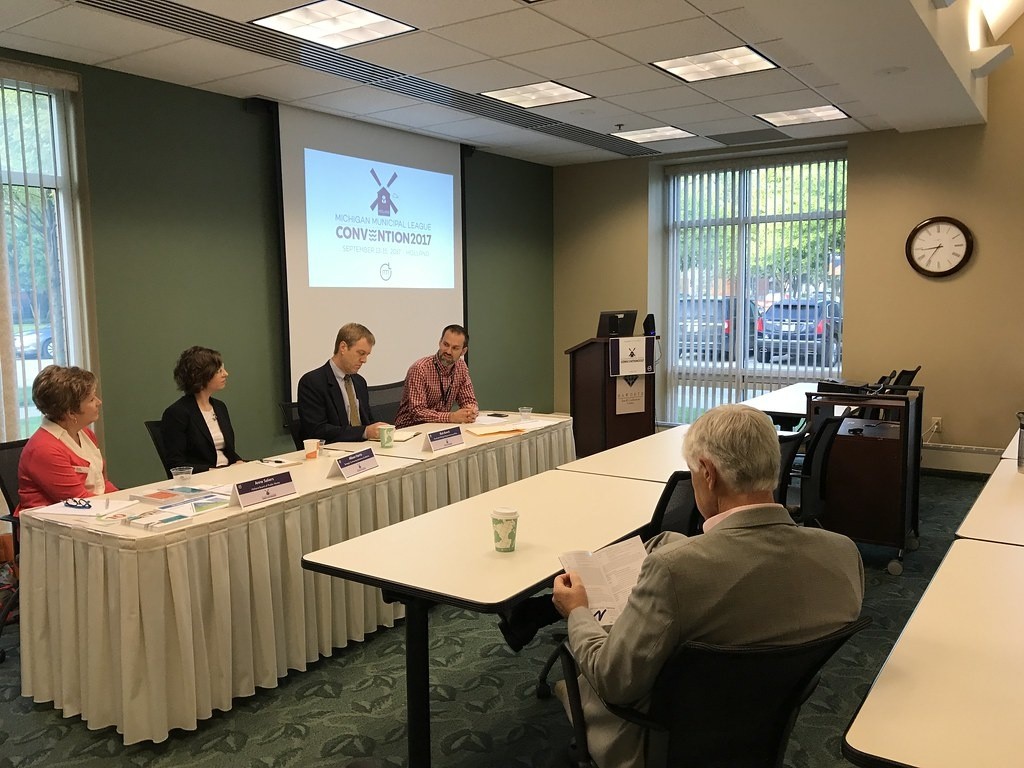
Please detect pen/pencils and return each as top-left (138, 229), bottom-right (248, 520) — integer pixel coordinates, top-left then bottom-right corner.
top-left (487, 413), bottom-right (508, 417)
top-left (105, 498), bottom-right (109, 511)
top-left (260, 459), bottom-right (285, 463)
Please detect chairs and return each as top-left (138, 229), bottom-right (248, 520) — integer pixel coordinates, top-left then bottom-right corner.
top-left (772, 421), bottom-right (814, 508)
top-left (144, 420), bottom-right (173, 480)
top-left (278, 401), bottom-right (304, 451)
top-left (790, 407), bottom-right (851, 529)
top-left (880, 365), bottom-right (922, 394)
top-left (367, 380), bottom-right (405, 425)
top-left (535, 616), bottom-right (877, 768)
top-left (648, 471), bottom-right (698, 539)
top-left (0, 439), bottom-right (31, 664)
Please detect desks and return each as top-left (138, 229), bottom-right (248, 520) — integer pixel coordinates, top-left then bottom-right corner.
top-left (842, 428), bottom-right (1024, 767)
top-left (299, 383), bottom-right (889, 768)
top-left (18, 410), bottom-right (577, 746)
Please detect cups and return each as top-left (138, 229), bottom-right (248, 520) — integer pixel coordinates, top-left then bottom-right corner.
top-left (519, 407), bottom-right (533, 423)
top-left (303, 439), bottom-right (320, 461)
top-left (378, 425), bottom-right (395, 448)
top-left (170, 467), bottom-right (193, 487)
top-left (319, 440), bottom-right (325, 456)
top-left (1017, 412), bottom-right (1024, 474)
top-left (491, 507), bottom-right (519, 552)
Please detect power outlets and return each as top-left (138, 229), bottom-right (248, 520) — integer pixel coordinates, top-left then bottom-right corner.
top-left (931, 417), bottom-right (942, 432)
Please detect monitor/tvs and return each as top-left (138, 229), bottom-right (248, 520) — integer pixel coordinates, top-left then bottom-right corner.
top-left (596, 310), bottom-right (638, 338)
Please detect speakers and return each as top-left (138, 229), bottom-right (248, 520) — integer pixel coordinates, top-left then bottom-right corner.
top-left (643, 314), bottom-right (655, 336)
top-left (608, 315), bottom-right (618, 338)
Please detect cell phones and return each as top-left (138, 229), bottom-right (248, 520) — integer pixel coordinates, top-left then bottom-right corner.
top-left (487, 413), bottom-right (508, 417)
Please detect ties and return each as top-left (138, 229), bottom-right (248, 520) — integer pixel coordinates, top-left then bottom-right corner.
top-left (344, 374), bottom-right (360, 426)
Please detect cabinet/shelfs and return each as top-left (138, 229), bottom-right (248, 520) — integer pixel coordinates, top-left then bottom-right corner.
top-left (805, 384), bottom-right (924, 576)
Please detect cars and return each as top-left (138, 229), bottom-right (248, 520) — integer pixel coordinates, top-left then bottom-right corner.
top-left (14, 326), bottom-right (52, 361)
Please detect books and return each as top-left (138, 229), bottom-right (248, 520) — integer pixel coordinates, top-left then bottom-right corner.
top-left (368, 429), bottom-right (423, 442)
top-left (557, 534), bottom-right (648, 633)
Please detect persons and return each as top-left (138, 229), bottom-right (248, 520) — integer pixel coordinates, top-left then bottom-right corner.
top-left (297, 322), bottom-right (397, 452)
top-left (160, 345), bottom-right (246, 479)
top-left (552, 404), bottom-right (865, 768)
top-left (393, 324), bottom-right (479, 430)
top-left (12, 364), bottom-right (120, 544)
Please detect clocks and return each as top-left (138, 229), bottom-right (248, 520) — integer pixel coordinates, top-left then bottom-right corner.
top-left (905, 216), bottom-right (974, 279)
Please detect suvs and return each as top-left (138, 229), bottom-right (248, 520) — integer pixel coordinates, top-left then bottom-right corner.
top-left (678, 297), bottom-right (761, 359)
top-left (755, 300), bottom-right (843, 366)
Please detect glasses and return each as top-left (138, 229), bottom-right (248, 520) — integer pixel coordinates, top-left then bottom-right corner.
top-left (65, 497), bottom-right (92, 509)
top-left (215, 363), bottom-right (225, 374)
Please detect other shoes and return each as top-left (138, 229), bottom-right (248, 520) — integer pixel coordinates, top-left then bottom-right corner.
top-left (499, 598), bottom-right (540, 652)
